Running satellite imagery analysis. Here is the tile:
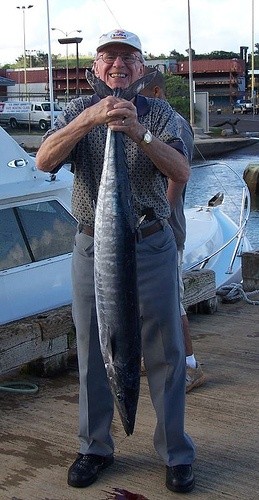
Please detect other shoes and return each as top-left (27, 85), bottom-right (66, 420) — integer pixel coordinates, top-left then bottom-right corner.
top-left (137, 364), bottom-right (148, 376)
top-left (181, 362), bottom-right (206, 394)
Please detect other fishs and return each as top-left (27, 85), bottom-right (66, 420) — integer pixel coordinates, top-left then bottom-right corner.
top-left (85, 69), bottom-right (159, 436)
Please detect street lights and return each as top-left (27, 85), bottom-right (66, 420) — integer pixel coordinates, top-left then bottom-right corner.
top-left (16, 5), bottom-right (34, 101)
top-left (51, 27), bottom-right (82, 102)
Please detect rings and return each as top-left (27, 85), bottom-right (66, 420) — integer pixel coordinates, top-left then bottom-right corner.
top-left (121, 120), bottom-right (125, 125)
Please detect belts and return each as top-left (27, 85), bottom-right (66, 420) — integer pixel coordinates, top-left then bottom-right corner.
top-left (82, 222), bottom-right (164, 240)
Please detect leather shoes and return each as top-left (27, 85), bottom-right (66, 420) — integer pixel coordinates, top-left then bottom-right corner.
top-left (166, 465), bottom-right (197, 493)
top-left (67, 453), bottom-right (115, 487)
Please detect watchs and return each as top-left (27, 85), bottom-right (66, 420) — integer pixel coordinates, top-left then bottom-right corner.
top-left (138, 129), bottom-right (153, 149)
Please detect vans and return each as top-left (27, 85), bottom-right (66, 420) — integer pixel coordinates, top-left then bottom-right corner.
top-left (0, 100), bottom-right (63, 130)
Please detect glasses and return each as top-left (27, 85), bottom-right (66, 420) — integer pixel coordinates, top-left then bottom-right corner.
top-left (96, 51), bottom-right (143, 66)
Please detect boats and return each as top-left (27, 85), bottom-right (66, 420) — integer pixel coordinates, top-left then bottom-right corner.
top-left (0, 128), bottom-right (256, 327)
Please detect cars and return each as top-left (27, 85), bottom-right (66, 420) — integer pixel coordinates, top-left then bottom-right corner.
top-left (232, 99), bottom-right (256, 115)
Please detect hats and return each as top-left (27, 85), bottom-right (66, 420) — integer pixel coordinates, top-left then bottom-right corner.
top-left (95, 29), bottom-right (148, 51)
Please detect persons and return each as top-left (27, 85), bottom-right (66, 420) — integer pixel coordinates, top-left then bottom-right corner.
top-left (35, 31), bottom-right (195, 494)
top-left (140, 67), bottom-right (208, 395)
